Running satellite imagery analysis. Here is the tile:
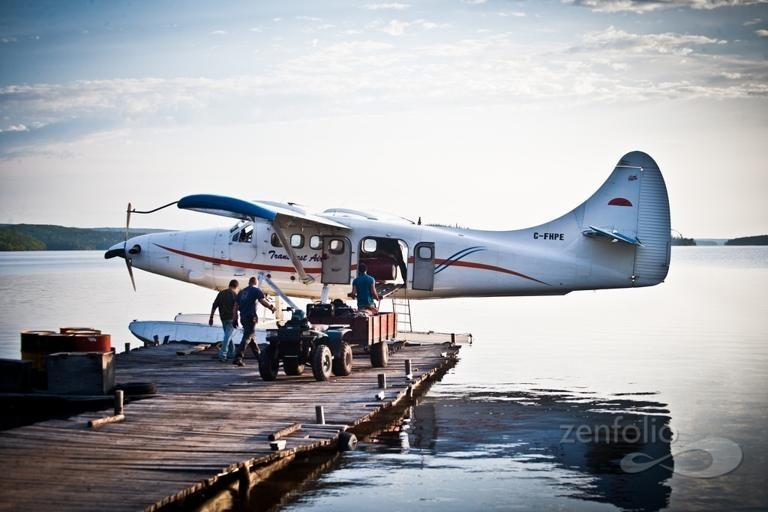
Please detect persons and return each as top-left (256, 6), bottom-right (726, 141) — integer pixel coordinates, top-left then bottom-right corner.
top-left (209, 280), bottom-right (239, 362)
top-left (351, 262), bottom-right (379, 309)
top-left (232, 277), bottom-right (277, 365)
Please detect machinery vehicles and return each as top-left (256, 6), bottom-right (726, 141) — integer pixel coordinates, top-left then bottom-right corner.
top-left (253, 299), bottom-right (399, 381)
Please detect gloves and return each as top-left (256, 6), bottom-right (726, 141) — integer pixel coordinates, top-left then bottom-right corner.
top-left (269, 304), bottom-right (277, 313)
top-left (232, 320), bottom-right (238, 328)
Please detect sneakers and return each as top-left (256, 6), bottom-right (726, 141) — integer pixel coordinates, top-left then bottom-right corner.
top-left (232, 361), bottom-right (245, 366)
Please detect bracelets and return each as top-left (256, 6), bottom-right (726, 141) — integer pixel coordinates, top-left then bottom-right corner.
top-left (270, 304), bottom-right (273, 309)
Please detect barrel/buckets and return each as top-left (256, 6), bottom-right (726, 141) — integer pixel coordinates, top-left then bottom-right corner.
top-left (20, 327), bottom-right (111, 391)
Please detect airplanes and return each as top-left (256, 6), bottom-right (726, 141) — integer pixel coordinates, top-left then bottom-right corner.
top-left (312, 378), bottom-right (673, 512)
top-left (102, 150), bottom-right (674, 348)
